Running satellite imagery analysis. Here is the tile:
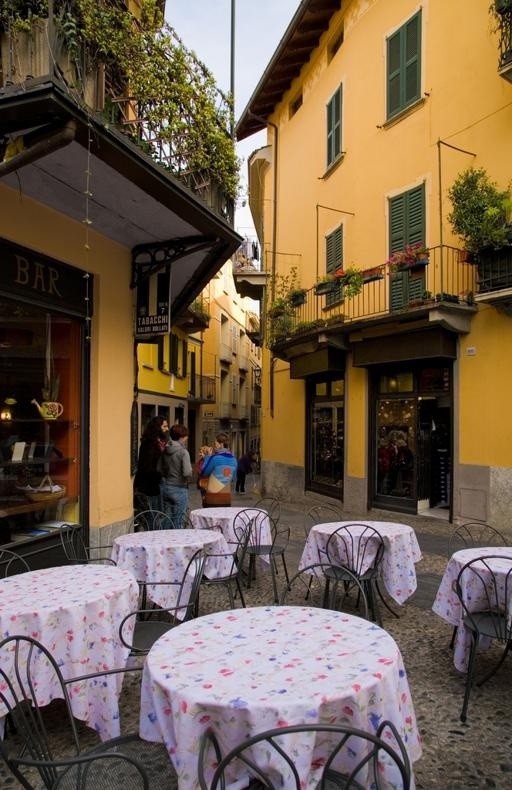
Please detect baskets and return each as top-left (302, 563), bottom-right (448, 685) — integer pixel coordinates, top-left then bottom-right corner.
top-left (26, 484), bottom-right (66, 503)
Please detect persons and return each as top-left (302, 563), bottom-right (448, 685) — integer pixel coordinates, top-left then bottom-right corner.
top-left (377, 440), bottom-right (414, 494)
top-left (234, 449), bottom-right (256, 496)
top-left (202, 433), bottom-right (238, 506)
top-left (156, 425), bottom-right (193, 529)
top-left (135, 415), bottom-right (168, 531)
top-left (198, 446), bottom-right (210, 498)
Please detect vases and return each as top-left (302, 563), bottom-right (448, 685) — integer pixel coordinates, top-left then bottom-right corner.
top-left (398, 252), bottom-right (429, 272)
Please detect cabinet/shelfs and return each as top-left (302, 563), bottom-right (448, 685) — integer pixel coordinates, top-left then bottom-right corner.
top-left (0, 417), bottom-right (81, 548)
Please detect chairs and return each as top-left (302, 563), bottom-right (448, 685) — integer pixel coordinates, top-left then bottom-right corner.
top-left (193, 519), bottom-right (254, 609)
top-left (3, 633), bottom-right (156, 787)
top-left (194, 718), bottom-right (414, 789)
top-left (59, 523), bottom-right (118, 567)
top-left (323, 525), bottom-right (399, 626)
top-left (116, 550), bottom-right (209, 654)
top-left (449, 521), bottom-right (508, 553)
top-left (304, 505), bottom-right (351, 598)
top-left (239, 496), bottom-right (283, 578)
top-left (454, 554), bottom-right (512, 721)
top-left (0, 549), bottom-right (32, 580)
top-left (127, 509), bottom-right (175, 534)
top-left (280, 563), bottom-right (370, 622)
top-left (233, 509), bottom-right (293, 602)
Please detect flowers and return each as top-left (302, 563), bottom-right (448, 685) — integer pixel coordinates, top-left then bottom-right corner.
top-left (380, 242), bottom-right (431, 279)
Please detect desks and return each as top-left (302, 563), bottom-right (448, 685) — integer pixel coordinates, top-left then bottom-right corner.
top-left (113, 529), bottom-right (239, 621)
top-left (299, 520), bottom-right (423, 605)
top-left (139, 606), bottom-right (422, 790)
top-left (0, 565), bottom-right (138, 745)
top-left (191, 506), bottom-right (274, 572)
top-left (430, 546), bottom-right (512, 677)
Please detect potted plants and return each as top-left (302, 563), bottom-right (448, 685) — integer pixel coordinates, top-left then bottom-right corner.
top-left (30, 352), bottom-right (65, 420)
top-left (268, 261), bottom-right (362, 342)
